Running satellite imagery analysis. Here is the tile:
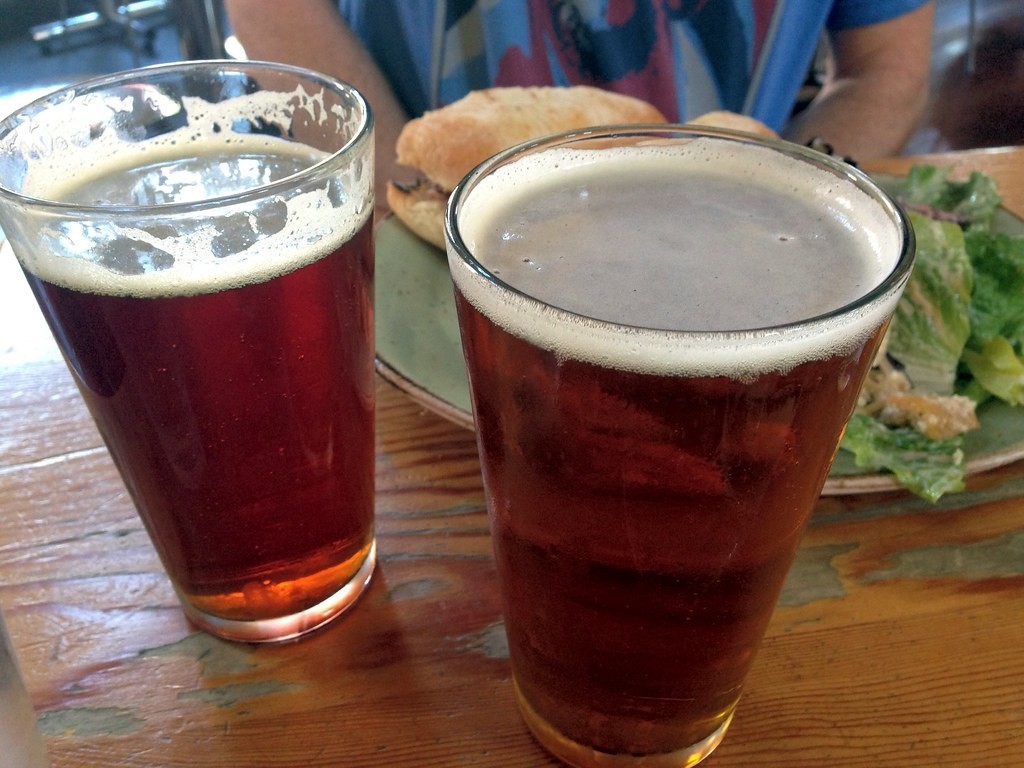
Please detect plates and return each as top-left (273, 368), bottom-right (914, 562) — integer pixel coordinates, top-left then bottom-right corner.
top-left (373, 168), bottom-right (1022, 500)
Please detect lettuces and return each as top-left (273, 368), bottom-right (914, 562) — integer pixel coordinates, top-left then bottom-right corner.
top-left (839, 148), bottom-right (1023, 506)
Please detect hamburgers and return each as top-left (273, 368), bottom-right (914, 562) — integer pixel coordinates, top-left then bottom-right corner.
top-left (379, 77), bottom-right (670, 256)
top-left (685, 109), bottom-right (782, 140)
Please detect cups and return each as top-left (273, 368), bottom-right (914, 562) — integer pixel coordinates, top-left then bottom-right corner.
top-left (446, 124), bottom-right (915, 768)
top-left (0, 57), bottom-right (381, 645)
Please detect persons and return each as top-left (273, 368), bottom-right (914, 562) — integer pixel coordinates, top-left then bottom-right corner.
top-left (927, 7), bottom-right (1024, 147)
top-left (211, 0), bottom-right (942, 265)
